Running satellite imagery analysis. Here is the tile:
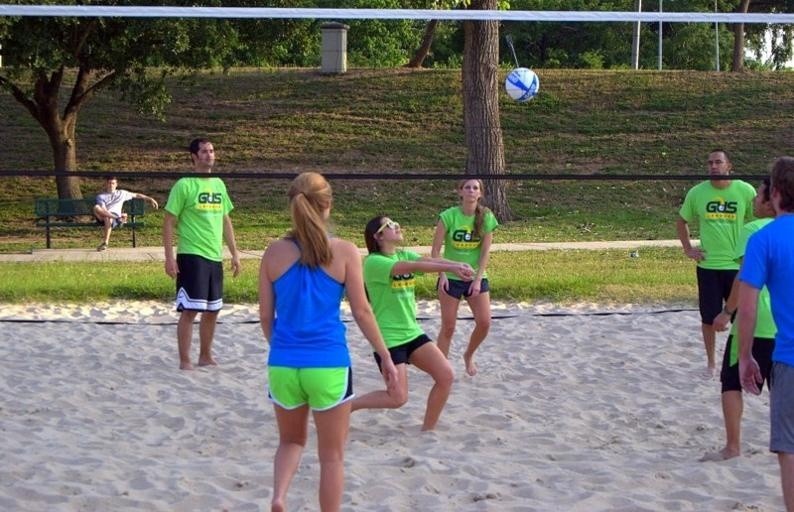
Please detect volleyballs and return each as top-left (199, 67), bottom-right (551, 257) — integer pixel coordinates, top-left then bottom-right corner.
top-left (505, 68), bottom-right (540, 102)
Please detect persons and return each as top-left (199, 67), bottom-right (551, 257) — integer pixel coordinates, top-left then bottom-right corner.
top-left (351, 215), bottom-right (476, 431)
top-left (92, 176), bottom-right (158, 251)
top-left (258, 171), bottom-right (399, 512)
top-left (432, 179), bottom-right (500, 376)
top-left (735, 158), bottom-right (793, 510)
top-left (702, 181), bottom-right (777, 462)
top-left (678, 149), bottom-right (760, 381)
top-left (163, 139), bottom-right (242, 370)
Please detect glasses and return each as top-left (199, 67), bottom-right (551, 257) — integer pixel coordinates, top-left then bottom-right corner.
top-left (375, 218), bottom-right (398, 232)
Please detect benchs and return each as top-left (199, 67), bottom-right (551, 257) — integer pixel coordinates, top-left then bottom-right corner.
top-left (33, 198), bottom-right (145, 249)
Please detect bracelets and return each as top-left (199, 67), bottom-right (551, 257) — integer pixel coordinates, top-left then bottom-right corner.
top-left (724, 305), bottom-right (733, 316)
